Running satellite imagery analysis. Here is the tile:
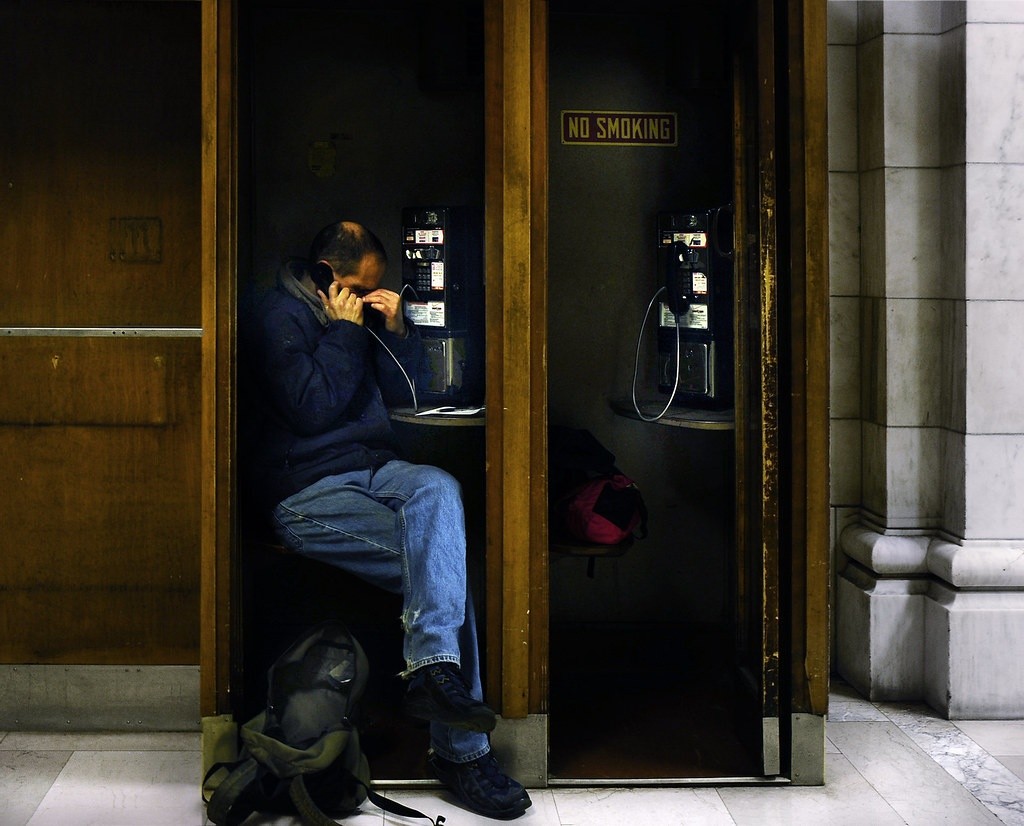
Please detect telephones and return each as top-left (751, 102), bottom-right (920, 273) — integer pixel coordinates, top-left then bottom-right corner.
top-left (652, 210), bottom-right (731, 342)
top-left (395, 210), bottom-right (478, 333)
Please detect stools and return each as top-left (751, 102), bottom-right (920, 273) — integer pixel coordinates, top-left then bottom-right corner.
top-left (550, 545), bottom-right (624, 557)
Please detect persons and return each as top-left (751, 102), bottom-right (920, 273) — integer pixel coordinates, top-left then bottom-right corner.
top-left (246, 223), bottom-right (532, 819)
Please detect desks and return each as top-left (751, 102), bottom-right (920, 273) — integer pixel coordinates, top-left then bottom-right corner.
top-left (614, 400), bottom-right (734, 430)
top-left (387, 404), bottom-right (487, 426)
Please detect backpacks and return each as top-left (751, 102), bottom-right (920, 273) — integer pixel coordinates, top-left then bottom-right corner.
top-left (202, 617), bottom-right (445, 826)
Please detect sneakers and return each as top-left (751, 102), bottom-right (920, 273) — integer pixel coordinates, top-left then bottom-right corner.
top-left (427, 747), bottom-right (531, 816)
top-left (403, 661), bottom-right (497, 734)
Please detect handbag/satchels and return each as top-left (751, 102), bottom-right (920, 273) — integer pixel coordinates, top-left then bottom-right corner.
top-left (568, 472), bottom-right (649, 545)
